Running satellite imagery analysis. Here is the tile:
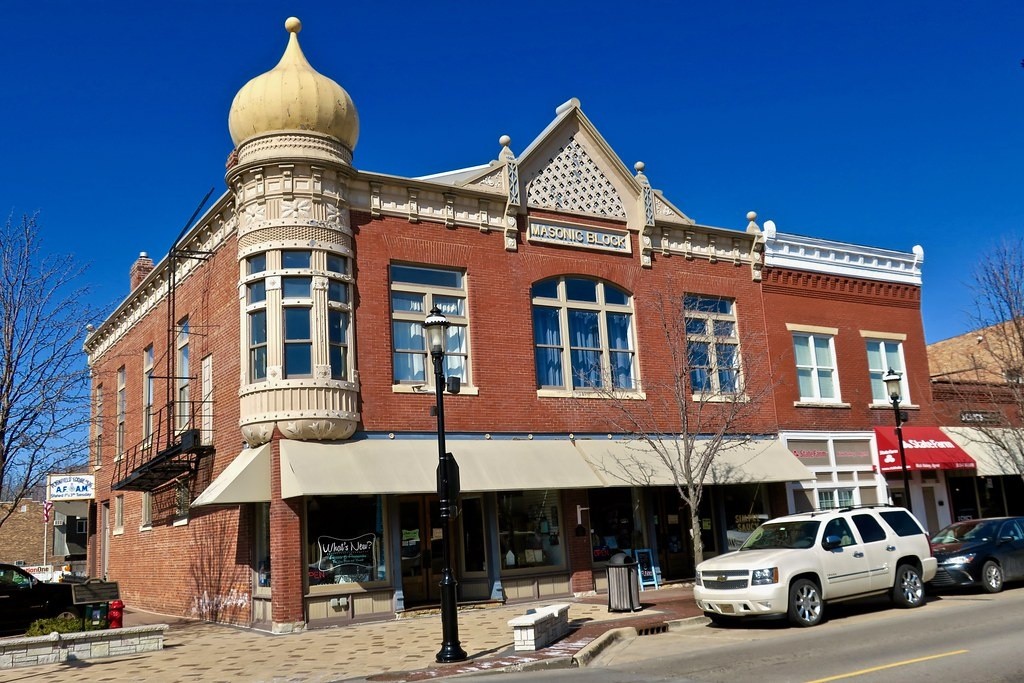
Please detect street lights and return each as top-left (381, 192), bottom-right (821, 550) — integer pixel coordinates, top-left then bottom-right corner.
top-left (422, 306), bottom-right (467, 663)
top-left (883, 366), bottom-right (913, 513)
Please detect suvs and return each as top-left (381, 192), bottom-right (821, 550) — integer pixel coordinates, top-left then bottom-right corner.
top-left (694, 504), bottom-right (939, 627)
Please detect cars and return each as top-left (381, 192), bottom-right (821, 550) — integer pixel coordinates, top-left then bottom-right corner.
top-left (1, 563), bottom-right (97, 638)
top-left (931, 514), bottom-right (1023, 593)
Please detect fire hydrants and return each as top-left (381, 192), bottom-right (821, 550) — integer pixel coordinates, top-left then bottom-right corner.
top-left (109, 600), bottom-right (126, 629)
